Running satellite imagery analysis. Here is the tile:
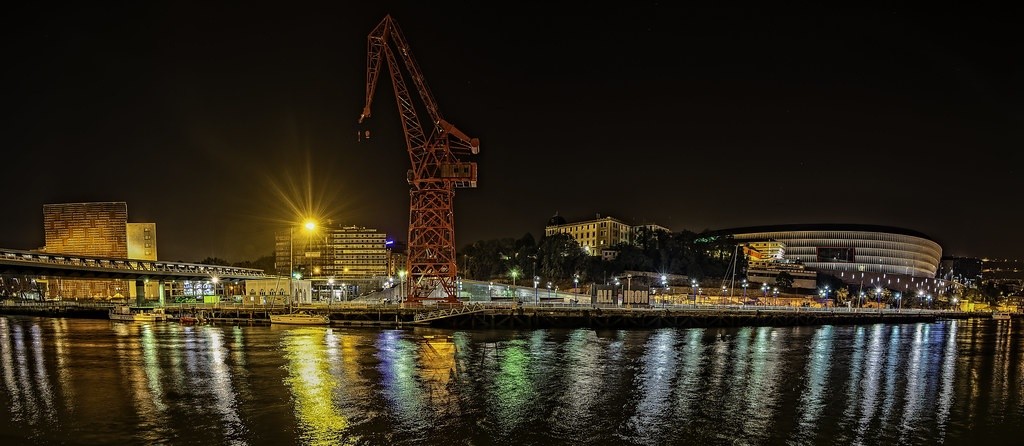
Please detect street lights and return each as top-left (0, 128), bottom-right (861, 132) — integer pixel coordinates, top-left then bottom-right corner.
top-left (761, 283), bottom-right (771, 310)
top-left (692, 279), bottom-right (697, 309)
top-left (512, 271), bottom-right (517, 307)
top-left (721, 285), bottom-right (728, 309)
top-left (876, 287), bottom-right (882, 312)
top-left (917, 290), bottom-right (925, 310)
top-left (860, 291), bottom-right (866, 308)
top-left (534, 280), bottom-right (539, 306)
top-left (926, 295), bottom-right (933, 310)
top-left (574, 278), bottom-right (579, 307)
top-left (339, 283), bottom-right (347, 302)
top-left (327, 279), bottom-right (335, 303)
top-left (742, 279), bottom-right (748, 306)
top-left (952, 297), bottom-right (958, 311)
top-left (398, 269), bottom-right (404, 307)
top-left (306, 222), bottom-right (316, 278)
top-left (213, 277), bottom-right (219, 297)
top-left (547, 285), bottom-right (551, 306)
top-left (660, 275), bottom-right (666, 310)
top-left (772, 286), bottom-right (780, 306)
top-left (894, 293), bottom-right (902, 313)
top-left (295, 274), bottom-right (302, 307)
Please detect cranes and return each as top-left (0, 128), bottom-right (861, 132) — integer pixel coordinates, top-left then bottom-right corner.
top-left (358, 12), bottom-right (480, 305)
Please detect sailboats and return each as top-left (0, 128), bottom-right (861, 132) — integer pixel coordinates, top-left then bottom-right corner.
top-left (268, 227), bottom-right (332, 326)
